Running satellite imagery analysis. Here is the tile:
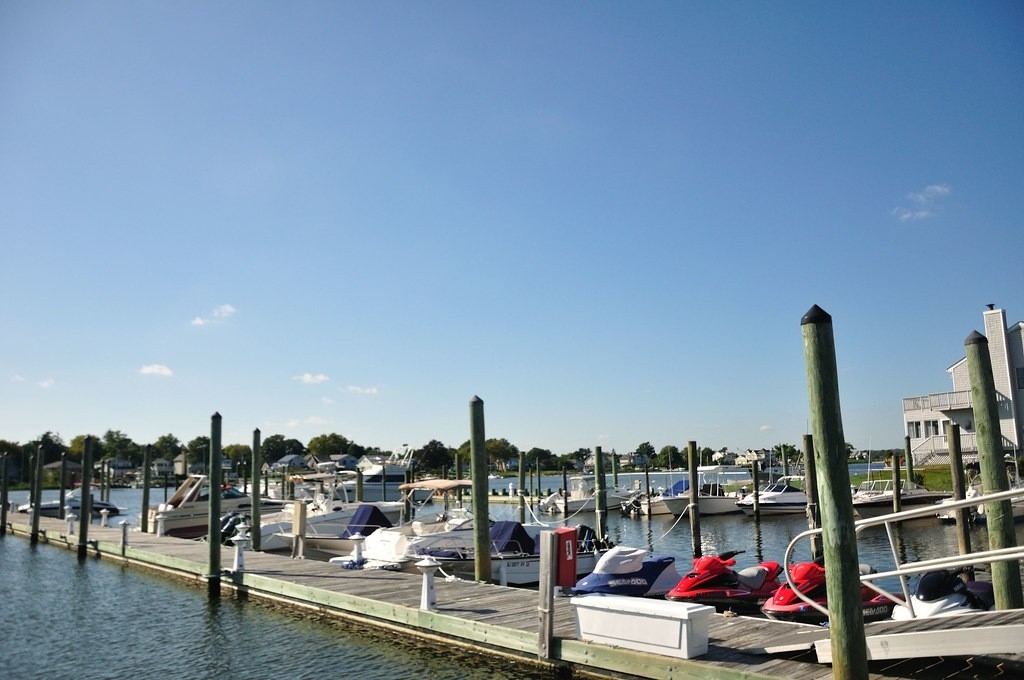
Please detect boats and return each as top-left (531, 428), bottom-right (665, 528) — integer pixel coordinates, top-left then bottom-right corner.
top-left (16, 488), bottom-right (120, 519)
top-left (149, 446), bottom-right (1024, 587)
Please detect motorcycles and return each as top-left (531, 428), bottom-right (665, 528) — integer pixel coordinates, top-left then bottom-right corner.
top-left (570, 546), bottom-right (686, 599)
top-left (761, 553), bottom-right (913, 626)
top-left (662, 549), bottom-right (785, 617)
top-left (889, 565), bottom-right (998, 622)
